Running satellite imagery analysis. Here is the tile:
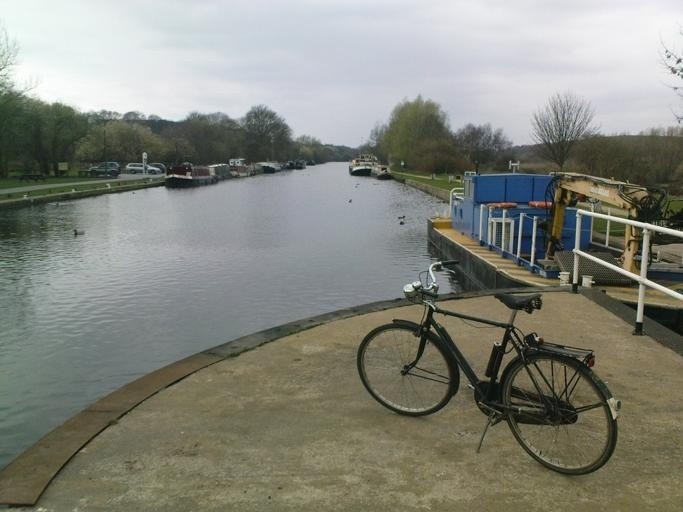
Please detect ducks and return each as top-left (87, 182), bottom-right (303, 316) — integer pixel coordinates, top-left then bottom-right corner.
top-left (73, 228), bottom-right (85, 235)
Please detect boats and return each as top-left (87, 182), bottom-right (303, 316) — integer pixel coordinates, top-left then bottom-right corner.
top-left (424, 167), bottom-right (682, 331)
top-left (346, 151), bottom-right (395, 181)
top-left (165, 155), bottom-right (317, 186)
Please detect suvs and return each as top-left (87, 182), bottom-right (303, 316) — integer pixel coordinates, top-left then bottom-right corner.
top-left (88, 161), bottom-right (123, 179)
top-left (148, 162), bottom-right (164, 174)
top-left (125, 161), bottom-right (160, 175)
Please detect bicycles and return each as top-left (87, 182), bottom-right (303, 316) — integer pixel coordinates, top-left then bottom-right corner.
top-left (352, 257), bottom-right (625, 477)
